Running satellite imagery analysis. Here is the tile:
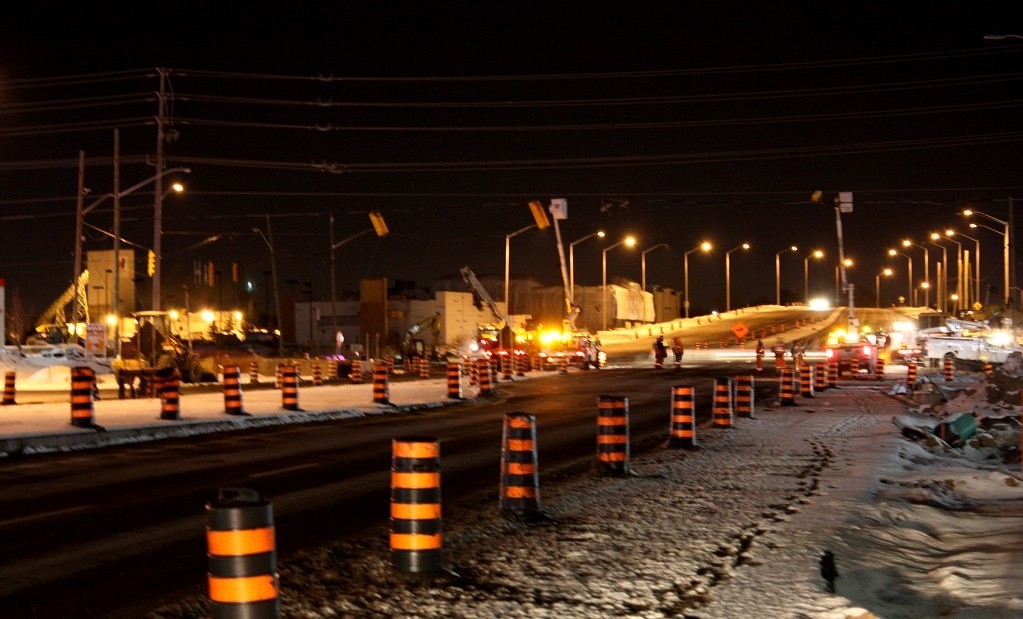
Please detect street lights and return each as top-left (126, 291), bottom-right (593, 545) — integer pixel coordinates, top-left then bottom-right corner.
top-left (775, 246), bottom-right (797, 304)
top-left (684, 240), bottom-right (712, 317)
top-left (835, 257), bottom-right (854, 306)
top-left (876, 267), bottom-right (893, 308)
top-left (727, 243), bottom-right (750, 312)
top-left (888, 248), bottom-right (914, 307)
top-left (570, 231), bottom-right (606, 305)
top-left (602, 235), bottom-right (636, 332)
top-left (804, 249), bottom-right (823, 305)
top-left (902, 239), bottom-right (930, 307)
top-left (113, 127), bottom-right (192, 358)
top-left (930, 209), bottom-right (1010, 315)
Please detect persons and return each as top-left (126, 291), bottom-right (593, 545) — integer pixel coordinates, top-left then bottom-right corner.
top-left (672, 337), bottom-right (683, 362)
top-left (770, 342), bottom-right (786, 368)
top-left (594, 338), bottom-right (601, 350)
top-left (883, 332), bottom-right (891, 348)
top-left (756, 340), bottom-right (764, 371)
top-left (858, 333), bottom-right (869, 342)
top-left (53, 328), bottom-right (61, 342)
top-left (655, 336), bottom-right (667, 365)
top-left (791, 340), bottom-right (806, 367)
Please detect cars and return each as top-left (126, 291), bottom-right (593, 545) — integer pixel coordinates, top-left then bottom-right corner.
top-left (826, 328), bottom-right (891, 375)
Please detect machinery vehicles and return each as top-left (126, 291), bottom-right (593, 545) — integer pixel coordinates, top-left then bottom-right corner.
top-left (111, 309), bottom-right (194, 399)
top-left (376, 311), bottom-right (460, 365)
